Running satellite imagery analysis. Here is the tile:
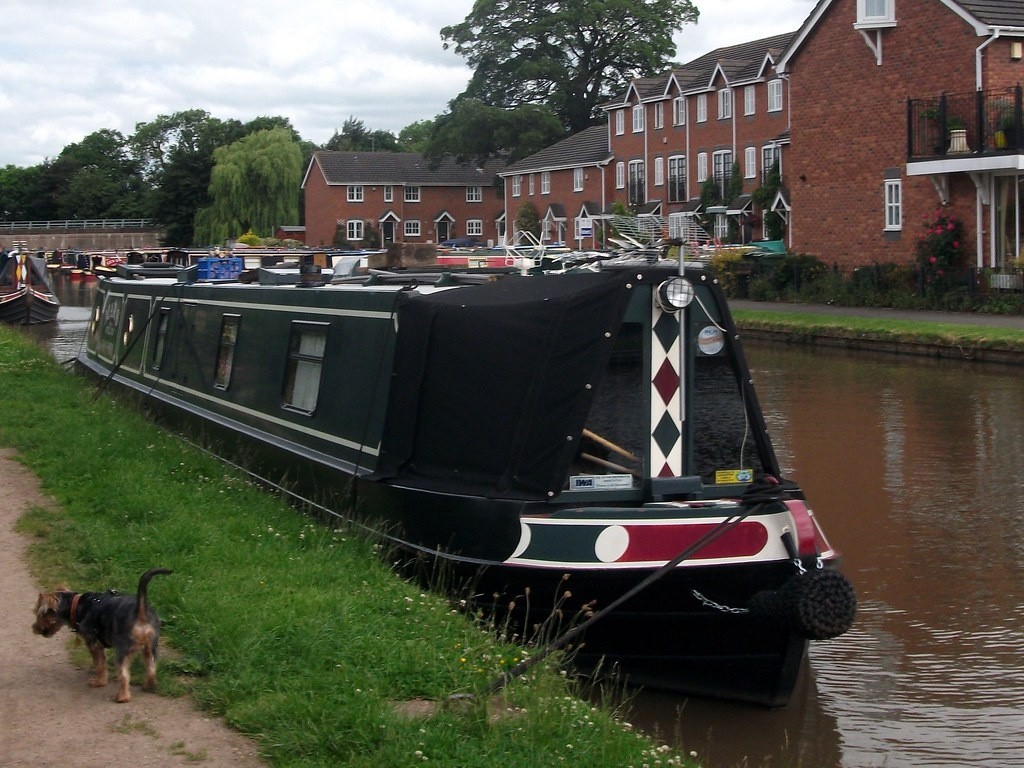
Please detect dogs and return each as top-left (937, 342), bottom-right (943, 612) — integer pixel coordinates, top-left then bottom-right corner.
top-left (30, 568), bottom-right (174, 703)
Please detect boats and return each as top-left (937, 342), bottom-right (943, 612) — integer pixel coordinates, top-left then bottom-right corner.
top-left (437, 242), bottom-right (572, 273)
top-left (72, 245), bottom-right (859, 712)
top-left (0, 242), bottom-right (61, 326)
top-left (10, 246), bottom-right (385, 281)
